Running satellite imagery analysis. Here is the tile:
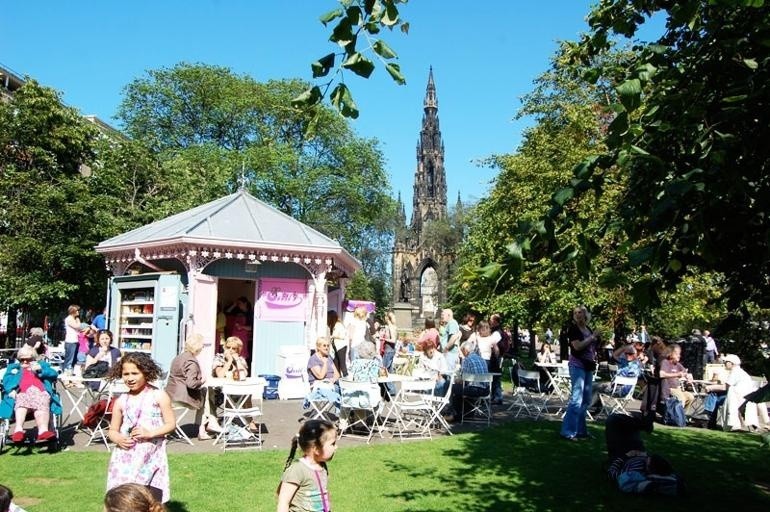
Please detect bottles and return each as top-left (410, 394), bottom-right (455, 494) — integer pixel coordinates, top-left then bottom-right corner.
top-left (238, 368), bottom-right (246, 380)
top-left (118, 290), bottom-right (154, 354)
top-left (226, 368), bottom-right (234, 381)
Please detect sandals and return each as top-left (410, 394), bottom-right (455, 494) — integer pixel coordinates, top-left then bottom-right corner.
top-left (561, 434), bottom-right (578, 442)
top-left (578, 432), bottom-right (596, 440)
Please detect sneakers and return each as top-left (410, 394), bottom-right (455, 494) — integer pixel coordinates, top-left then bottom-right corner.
top-left (198, 433), bottom-right (215, 440)
top-left (13, 431), bottom-right (24, 442)
top-left (207, 424), bottom-right (228, 434)
top-left (37, 431), bottom-right (56, 440)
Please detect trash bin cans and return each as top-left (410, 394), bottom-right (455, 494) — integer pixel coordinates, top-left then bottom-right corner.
top-left (258, 374), bottom-right (281, 400)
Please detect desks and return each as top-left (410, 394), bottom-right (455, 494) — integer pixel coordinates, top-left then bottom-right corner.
top-left (58, 374), bottom-right (268, 439)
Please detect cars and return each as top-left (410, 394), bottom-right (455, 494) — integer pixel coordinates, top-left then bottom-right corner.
top-left (0, 337), bottom-right (65, 365)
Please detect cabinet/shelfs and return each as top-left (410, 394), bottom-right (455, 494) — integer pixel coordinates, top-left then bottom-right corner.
top-left (118, 301), bottom-right (154, 355)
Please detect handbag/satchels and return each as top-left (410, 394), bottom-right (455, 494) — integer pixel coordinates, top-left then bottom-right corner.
top-left (351, 359), bottom-right (379, 383)
top-left (83, 361), bottom-right (108, 378)
top-left (583, 360), bottom-right (596, 371)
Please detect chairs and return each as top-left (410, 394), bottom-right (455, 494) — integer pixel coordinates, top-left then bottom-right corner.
top-left (298, 354), bottom-right (493, 442)
top-left (506, 360), bottom-right (770, 430)
top-left (159, 370), bottom-right (194, 446)
top-left (85, 383), bottom-right (129, 453)
top-left (212, 383), bottom-right (264, 453)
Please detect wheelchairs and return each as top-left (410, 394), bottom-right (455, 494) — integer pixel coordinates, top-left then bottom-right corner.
top-left (0, 362), bottom-right (61, 454)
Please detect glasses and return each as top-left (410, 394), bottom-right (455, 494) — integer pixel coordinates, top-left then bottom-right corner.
top-left (19, 357), bottom-right (34, 363)
top-left (227, 346), bottom-right (238, 351)
top-left (625, 352), bottom-right (635, 356)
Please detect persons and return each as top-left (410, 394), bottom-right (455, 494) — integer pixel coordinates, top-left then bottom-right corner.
top-left (165, 297), bottom-right (257, 440)
top-left (2, 304), bottom-right (123, 443)
top-left (533, 344), bottom-right (559, 394)
top-left (607, 414), bottom-right (672, 496)
top-left (277, 420), bottom-right (337, 512)
top-left (105, 351), bottom-right (176, 512)
top-left (306, 304), bottom-right (509, 435)
top-left (1, 485), bottom-right (24, 512)
top-left (560, 304), bottom-right (605, 441)
top-left (586, 324), bottom-right (769, 434)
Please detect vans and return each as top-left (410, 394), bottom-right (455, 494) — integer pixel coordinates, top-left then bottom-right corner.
top-left (517, 331), bottom-right (539, 349)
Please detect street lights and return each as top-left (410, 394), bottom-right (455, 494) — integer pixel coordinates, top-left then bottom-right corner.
top-left (399, 270), bottom-right (412, 302)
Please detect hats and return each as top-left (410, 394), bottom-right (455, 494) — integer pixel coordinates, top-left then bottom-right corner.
top-left (723, 354), bottom-right (741, 364)
